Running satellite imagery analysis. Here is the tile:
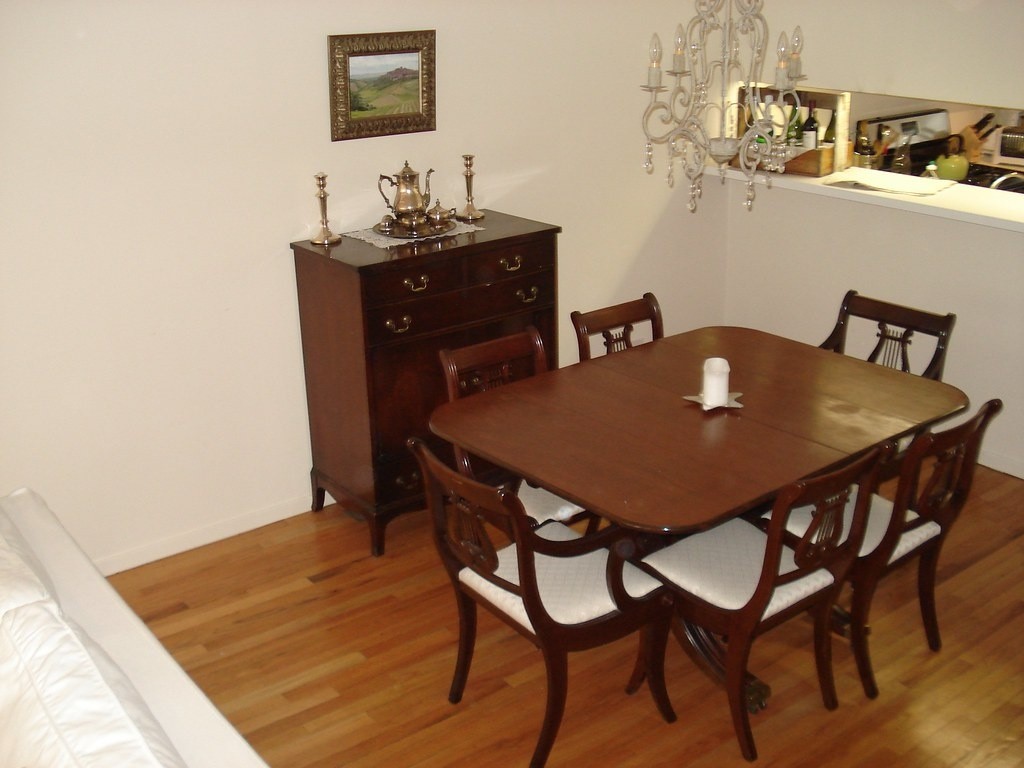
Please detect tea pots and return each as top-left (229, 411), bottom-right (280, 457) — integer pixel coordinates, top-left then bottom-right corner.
top-left (423, 200), bottom-right (457, 229)
top-left (378, 159), bottom-right (435, 222)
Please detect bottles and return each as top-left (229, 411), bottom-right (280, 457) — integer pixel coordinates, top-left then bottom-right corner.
top-left (848, 131), bottom-right (872, 169)
top-left (743, 90), bottom-right (837, 149)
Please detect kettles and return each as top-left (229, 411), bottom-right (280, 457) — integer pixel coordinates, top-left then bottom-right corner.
top-left (933, 133), bottom-right (970, 180)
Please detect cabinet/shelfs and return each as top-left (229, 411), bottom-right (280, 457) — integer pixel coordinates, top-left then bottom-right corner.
top-left (289, 209), bottom-right (560, 556)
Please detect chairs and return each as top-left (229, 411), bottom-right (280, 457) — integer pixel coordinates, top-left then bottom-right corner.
top-left (570, 292), bottom-right (663, 362)
top-left (408, 438), bottom-right (677, 768)
top-left (634, 442), bottom-right (899, 762)
top-left (440, 327), bottom-right (603, 538)
top-left (756, 399), bottom-right (1003, 700)
top-left (817, 290), bottom-right (957, 382)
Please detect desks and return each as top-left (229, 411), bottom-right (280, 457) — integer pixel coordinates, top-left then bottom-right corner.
top-left (436, 325), bottom-right (969, 726)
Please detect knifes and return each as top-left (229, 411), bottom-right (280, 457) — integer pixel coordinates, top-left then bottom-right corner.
top-left (971, 113), bottom-right (996, 141)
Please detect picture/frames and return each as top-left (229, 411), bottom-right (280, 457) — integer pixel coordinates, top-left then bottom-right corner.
top-left (328, 29), bottom-right (437, 141)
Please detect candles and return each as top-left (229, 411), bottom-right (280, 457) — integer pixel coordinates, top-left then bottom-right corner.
top-left (703, 358), bottom-right (730, 406)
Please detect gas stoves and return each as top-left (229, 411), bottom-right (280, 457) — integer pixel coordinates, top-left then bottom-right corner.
top-left (856, 108), bottom-right (1024, 194)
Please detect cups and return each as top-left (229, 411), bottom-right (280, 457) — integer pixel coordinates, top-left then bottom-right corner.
top-left (380, 215), bottom-right (394, 230)
top-left (702, 356), bottom-right (730, 408)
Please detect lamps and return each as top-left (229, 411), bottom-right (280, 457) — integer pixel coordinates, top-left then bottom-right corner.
top-left (640, 0), bottom-right (808, 212)
